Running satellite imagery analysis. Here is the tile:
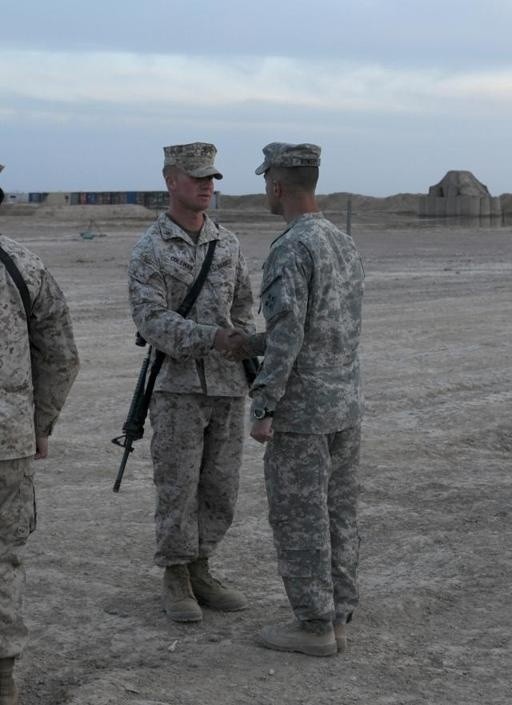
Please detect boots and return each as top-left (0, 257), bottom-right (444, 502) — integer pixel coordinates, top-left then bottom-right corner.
top-left (0, 659), bottom-right (18, 705)
top-left (189, 560), bottom-right (249, 611)
top-left (160, 565), bottom-right (201, 621)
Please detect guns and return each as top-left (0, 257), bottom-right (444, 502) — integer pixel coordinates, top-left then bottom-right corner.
top-left (111, 332), bottom-right (161, 492)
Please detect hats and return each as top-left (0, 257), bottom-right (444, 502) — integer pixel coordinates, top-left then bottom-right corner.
top-left (162, 142), bottom-right (222, 180)
top-left (255, 142), bottom-right (322, 176)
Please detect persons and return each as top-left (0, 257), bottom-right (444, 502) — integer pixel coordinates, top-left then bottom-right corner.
top-left (221, 142), bottom-right (366, 659)
top-left (0, 164), bottom-right (81, 704)
top-left (127, 140), bottom-right (260, 623)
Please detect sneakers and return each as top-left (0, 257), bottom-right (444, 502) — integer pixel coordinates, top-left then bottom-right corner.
top-left (252, 614), bottom-right (349, 656)
